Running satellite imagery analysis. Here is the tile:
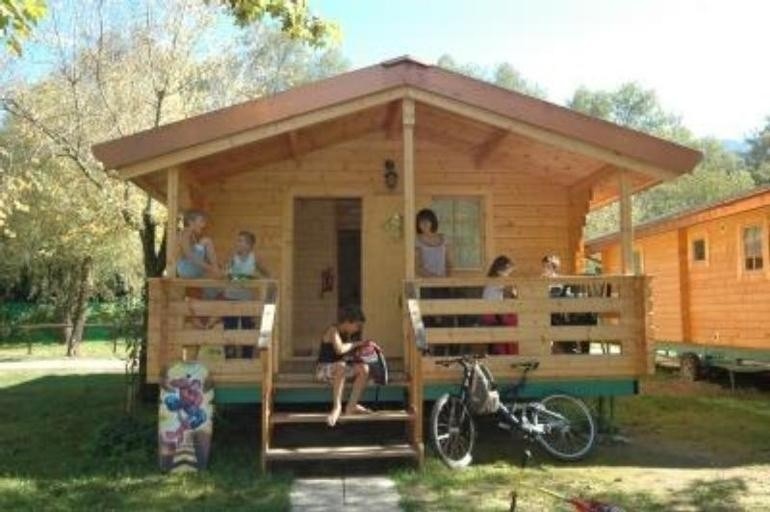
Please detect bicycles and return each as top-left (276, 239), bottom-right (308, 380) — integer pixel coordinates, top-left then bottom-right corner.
top-left (429, 353), bottom-right (596, 470)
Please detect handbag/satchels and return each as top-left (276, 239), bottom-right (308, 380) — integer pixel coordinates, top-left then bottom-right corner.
top-left (352, 344), bottom-right (389, 385)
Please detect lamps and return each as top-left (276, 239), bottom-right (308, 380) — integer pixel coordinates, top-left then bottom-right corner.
top-left (384, 159), bottom-right (398, 188)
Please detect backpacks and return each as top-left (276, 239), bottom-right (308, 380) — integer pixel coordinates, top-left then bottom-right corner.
top-left (469, 354), bottom-right (499, 414)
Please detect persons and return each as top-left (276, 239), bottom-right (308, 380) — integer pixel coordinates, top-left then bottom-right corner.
top-left (174, 206), bottom-right (222, 328)
top-left (313, 302), bottom-right (376, 428)
top-left (416, 206), bottom-right (458, 356)
top-left (478, 253), bottom-right (522, 354)
top-left (543, 254), bottom-right (591, 352)
top-left (223, 231), bottom-right (270, 359)
